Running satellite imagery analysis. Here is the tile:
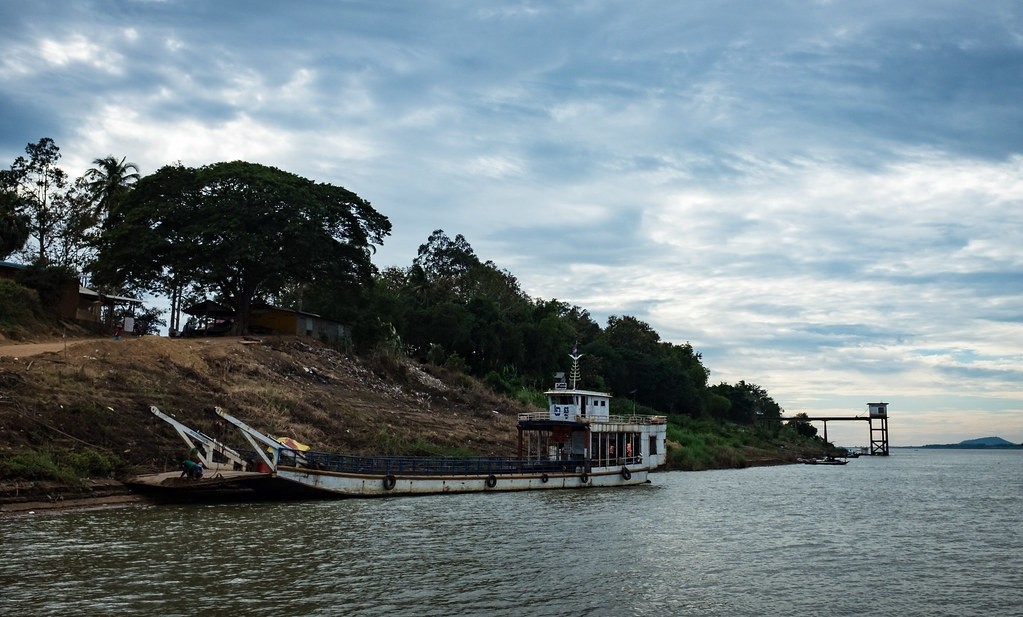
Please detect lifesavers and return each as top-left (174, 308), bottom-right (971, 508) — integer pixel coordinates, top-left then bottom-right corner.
top-left (542, 474), bottom-right (548, 483)
top-left (581, 473), bottom-right (587, 483)
top-left (621, 467), bottom-right (631, 480)
top-left (487, 475), bottom-right (496, 488)
top-left (383, 474), bottom-right (396, 490)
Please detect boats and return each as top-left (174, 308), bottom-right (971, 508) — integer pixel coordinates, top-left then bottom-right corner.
top-left (119, 340), bottom-right (667, 504)
top-left (801, 454), bottom-right (849, 465)
top-left (846, 450), bottom-right (862, 458)
